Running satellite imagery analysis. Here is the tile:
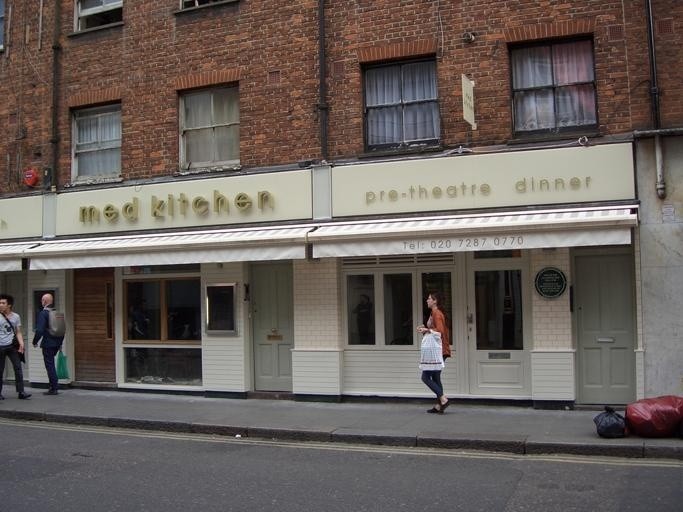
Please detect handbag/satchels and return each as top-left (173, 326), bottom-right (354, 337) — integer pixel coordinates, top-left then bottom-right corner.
top-left (11, 338), bottom-right (26, 365)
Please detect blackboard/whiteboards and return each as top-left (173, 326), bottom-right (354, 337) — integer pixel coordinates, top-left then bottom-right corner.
top-left (204, 283), bottom-right (236, 334)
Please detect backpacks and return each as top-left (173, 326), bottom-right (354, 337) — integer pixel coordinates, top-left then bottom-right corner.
top-left (44, 307), bottom-right (66, 338)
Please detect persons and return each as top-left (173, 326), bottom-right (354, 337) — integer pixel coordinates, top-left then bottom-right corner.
top-left (352, 294), bottom-right (374, 344)
top-left (0, 295), bottom-right (32, 400)
top-left (32, 293), bottom-right (65, 395)
top-left (131, 297), bottom-right (148, 337)
top-left (416, 292), bottom-right (451, 414)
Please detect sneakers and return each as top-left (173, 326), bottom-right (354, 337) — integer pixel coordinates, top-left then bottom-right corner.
top-left (440, 401), bottom-right (449, 413)
top-left (427, 408), bottom-right (443, 413)
top-left (44, 390), bottom-right (57, 395)
top-left (18, 392), bottom-right (31, 398)
